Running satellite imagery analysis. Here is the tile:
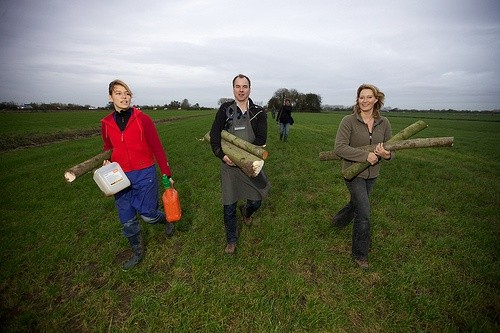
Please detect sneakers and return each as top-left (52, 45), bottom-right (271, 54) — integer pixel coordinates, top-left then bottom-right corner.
top-left (225, 243), bottom-right (235, 253)
top-left (239, 205), bottom-right (252, 225)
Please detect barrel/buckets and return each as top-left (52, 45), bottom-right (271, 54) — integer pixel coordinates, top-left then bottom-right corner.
top-left (92, 162), bottom-right (131, 197)
top-left (162, 174), bottom-right (181, 222)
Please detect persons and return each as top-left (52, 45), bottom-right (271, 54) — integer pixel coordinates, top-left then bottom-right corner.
top-left (330, 84), bottom-right (395, 268)
top-left (101, 80), bottom-right (175, 271)
top-left (271, 105), bottom-right (276, 119)
top-left (209, 74), bottom-right (268, 255)
top-left (276, 98), bottom-right (292, 145)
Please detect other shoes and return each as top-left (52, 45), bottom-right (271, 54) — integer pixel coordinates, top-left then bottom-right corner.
top-left (332, 221), bottom-right (349, 231)
top-left (353, 256), bottom-right (369, 269)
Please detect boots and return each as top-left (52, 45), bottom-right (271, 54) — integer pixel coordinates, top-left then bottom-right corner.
top-left (159, 215), bottom-right (174, 237)
top-left (122, 230), bottom-right (144, 272)
